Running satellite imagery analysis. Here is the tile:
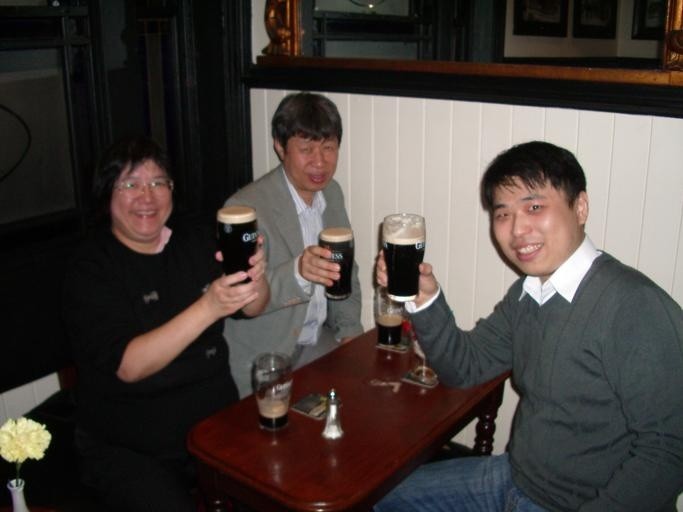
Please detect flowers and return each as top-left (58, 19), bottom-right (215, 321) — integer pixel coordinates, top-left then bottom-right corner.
top-left (0, 413), bottom-right (52, 487)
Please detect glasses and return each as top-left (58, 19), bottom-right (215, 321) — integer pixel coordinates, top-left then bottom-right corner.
top-left (111, 176), bottom-right (174, 193)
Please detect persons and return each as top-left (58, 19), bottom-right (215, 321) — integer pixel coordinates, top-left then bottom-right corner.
top-left (224, 93), bottom-right (363, 398)
top-left (52, 138), bottom-right (272, 511)
top-left (376, 142), bottom-right (682, 512)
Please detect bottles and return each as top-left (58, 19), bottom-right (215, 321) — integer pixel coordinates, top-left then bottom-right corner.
top-left (320, 388), bottom-right (345, 440)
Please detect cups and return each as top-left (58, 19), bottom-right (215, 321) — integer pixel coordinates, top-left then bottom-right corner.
top-left (215, 206), bottom-right (257, 287)
top-left (408, 331), bottom-right (437, 385)
top-left (251, 350), bottom-right (290, 432)
top-left (378, 214), bottom-right (426, 303)
top-left (319, 228), bottom-right (354, 302)
top-left (373, 292), bottom-right (404, 346)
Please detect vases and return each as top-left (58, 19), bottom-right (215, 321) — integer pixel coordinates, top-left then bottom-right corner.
top-left (6, 478), bottom-right (31, 512)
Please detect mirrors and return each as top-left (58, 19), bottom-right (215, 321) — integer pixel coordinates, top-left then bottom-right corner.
top-left (239, 1), bottom-right (683, 118)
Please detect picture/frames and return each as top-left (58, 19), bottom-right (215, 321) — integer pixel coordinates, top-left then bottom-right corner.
top-left (572, 0), bottom-right (617, 37)
top-left (512, 0), bottom-right (568, 36)
top-left (631, 0), bottom-right (666, 40)
